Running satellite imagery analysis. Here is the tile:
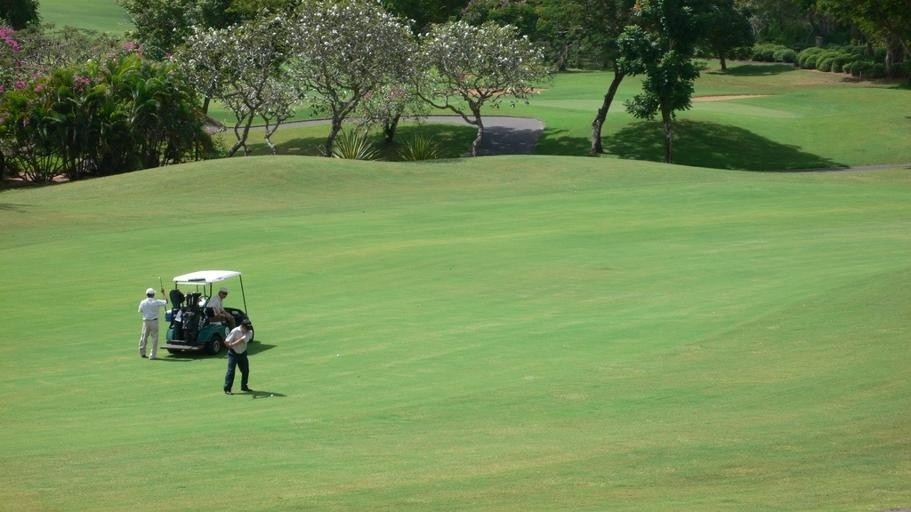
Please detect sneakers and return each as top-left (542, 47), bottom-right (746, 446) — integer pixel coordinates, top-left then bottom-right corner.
top-left (242, 388), bottom-right (252, 391)
top-left (225, 391), bottom-right (233, 395)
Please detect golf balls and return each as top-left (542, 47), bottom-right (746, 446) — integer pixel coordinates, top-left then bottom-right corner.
top-left (270, 394), bottom-right (274, 397)
top-left (336, 353), bottom-right (340, 356)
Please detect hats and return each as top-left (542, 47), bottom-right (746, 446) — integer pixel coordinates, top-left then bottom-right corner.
top-left (242, 320), bottom-right (253, 331)
top-left (146, 289), bottom-right (156, 296)
top-left (219, 287), bottom-right (229, 293)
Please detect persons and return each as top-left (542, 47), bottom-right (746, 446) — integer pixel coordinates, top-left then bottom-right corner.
top-left (138, 287), bottom-right (169, 361)
top-left (223, 317), bottom-right (254, 395)
top-left (205, 287), bottom-right (237, 329)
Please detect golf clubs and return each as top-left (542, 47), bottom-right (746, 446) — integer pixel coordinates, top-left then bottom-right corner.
top-left (158, 276), bottom-right (167, 311)
top-left (225, 327), bottom-right (231, 344)
top-left (184, 293), bottom-right (201, 306)
